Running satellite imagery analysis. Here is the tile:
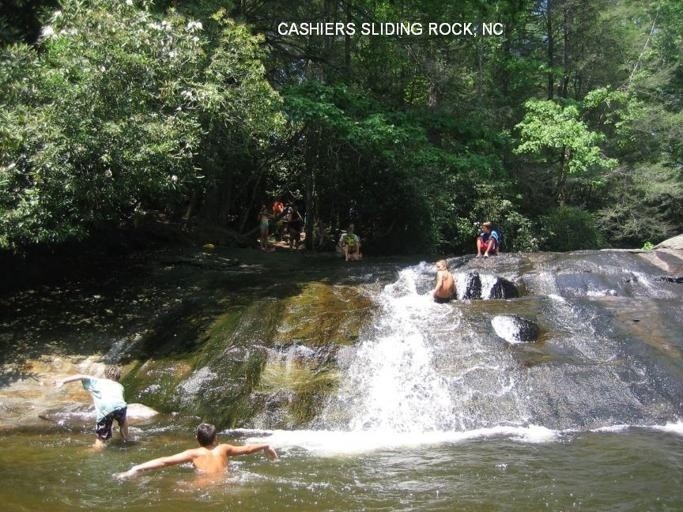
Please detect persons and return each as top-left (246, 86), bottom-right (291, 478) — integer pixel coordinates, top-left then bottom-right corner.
top-left (50, 365), bottom-right (129, 454)
top-left (337, 224), bottom-right (363, 262)
top-left (114, 422), bottom-right (279, 489)
top-left (433, 258), bottom-right (458, 304)
top-left (476, 221), bottom-right (498, 258)
top-left (258, 199), bottom-right (305, 252)
